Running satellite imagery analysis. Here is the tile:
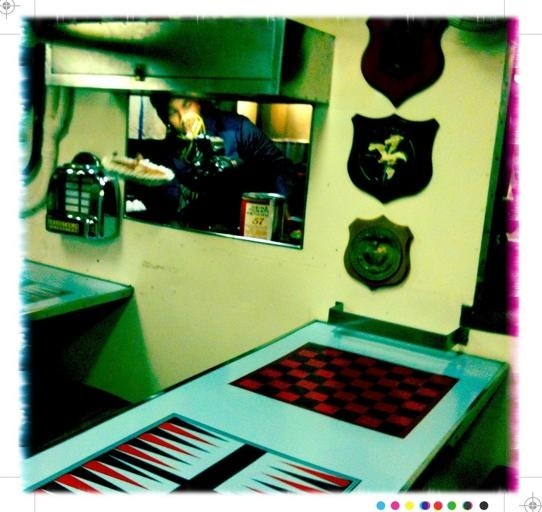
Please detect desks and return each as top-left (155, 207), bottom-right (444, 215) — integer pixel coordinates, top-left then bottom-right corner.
top-left (22, 257), bottom-right (133, 326)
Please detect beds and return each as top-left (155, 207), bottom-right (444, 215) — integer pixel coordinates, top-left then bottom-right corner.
top-left (22, 319), bottom-right (510, 493)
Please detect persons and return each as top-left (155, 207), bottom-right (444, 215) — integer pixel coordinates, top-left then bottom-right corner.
top-left (151, 95), bottom-right (290, 228)
